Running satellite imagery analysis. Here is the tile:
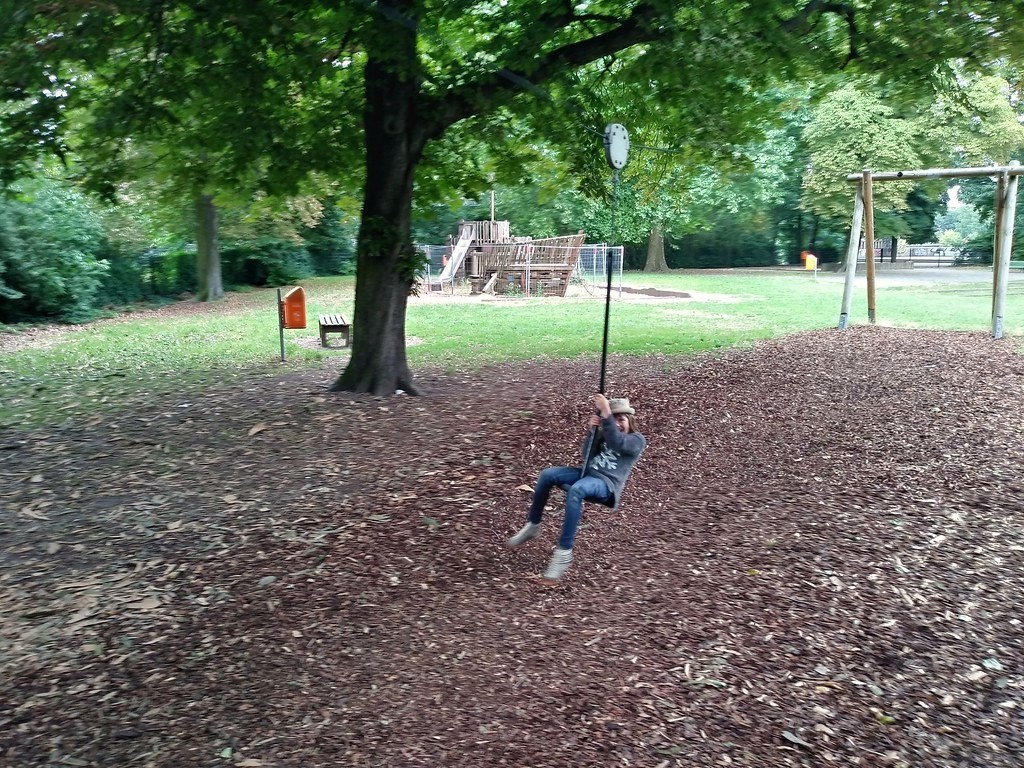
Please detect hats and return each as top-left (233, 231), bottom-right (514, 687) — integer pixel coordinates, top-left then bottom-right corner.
top-left (608, 399), bottom-right (634, 414)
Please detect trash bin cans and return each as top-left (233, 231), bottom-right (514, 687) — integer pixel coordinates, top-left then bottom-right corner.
top-left (281, 284), bottom-right (308, 329)
top-left (806, 254), bottom-right (816, 270)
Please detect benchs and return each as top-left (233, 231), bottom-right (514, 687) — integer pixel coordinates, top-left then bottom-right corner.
top-left (989, 261), bottom-right (1024, 272)
top-left (318, 313), bottom-right (352, 347)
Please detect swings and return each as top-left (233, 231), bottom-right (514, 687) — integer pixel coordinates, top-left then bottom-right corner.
top-left (556, 167), bottom-right (620, 509)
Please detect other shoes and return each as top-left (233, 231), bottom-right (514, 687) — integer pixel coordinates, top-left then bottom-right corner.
top-left (545, 549), bottom-right (573, 581)
top-left (507, 522), bottom-right (539, 547)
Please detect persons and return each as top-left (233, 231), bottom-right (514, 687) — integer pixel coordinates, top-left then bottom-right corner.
top-left (508, 395), bottom-right (644, 580)
